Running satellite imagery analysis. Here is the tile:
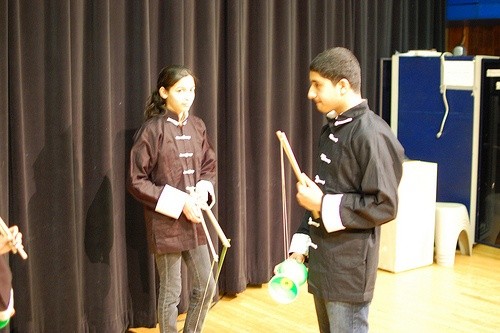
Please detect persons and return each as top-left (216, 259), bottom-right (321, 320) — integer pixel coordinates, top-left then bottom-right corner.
top-left (294, 47), bottom-right (403, 333)
top-left (125, 64), bottom-right (218, 333)
top-left (0, 215), bottom-right (25, 333)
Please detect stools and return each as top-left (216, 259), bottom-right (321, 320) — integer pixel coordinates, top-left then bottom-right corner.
top-left (435, 201), bottom-right (472, 265)
